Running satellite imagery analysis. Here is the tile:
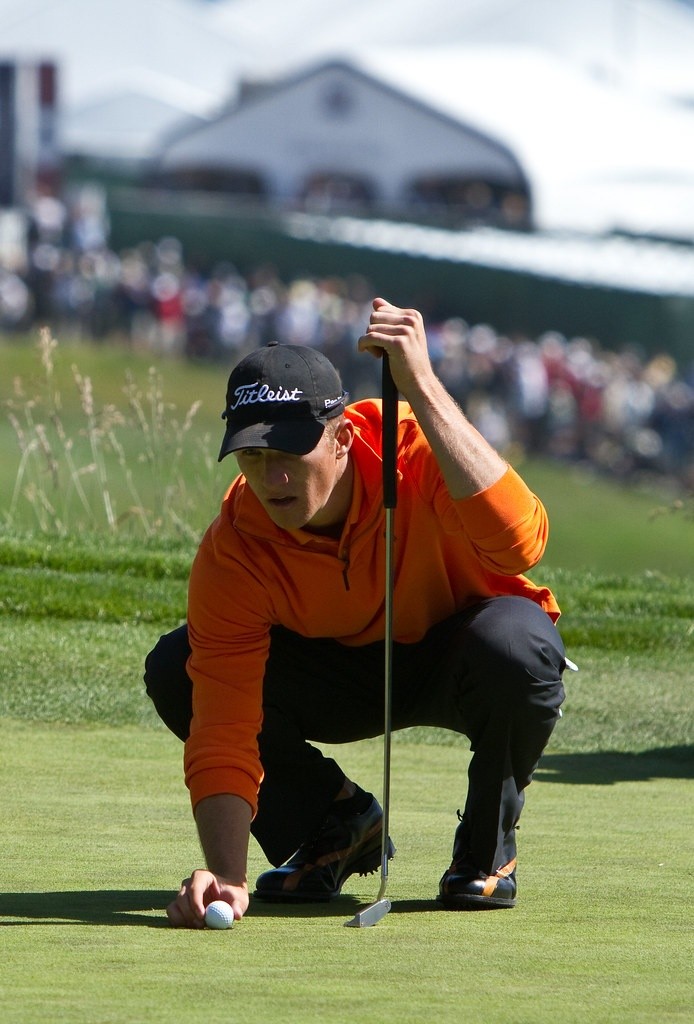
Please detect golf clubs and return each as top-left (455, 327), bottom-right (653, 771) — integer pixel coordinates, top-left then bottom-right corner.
top-left (346, 348), bottom-right (401, 928)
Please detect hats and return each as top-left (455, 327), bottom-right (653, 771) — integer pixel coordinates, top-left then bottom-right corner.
top-left (217, 341), bottom-right (349, 461)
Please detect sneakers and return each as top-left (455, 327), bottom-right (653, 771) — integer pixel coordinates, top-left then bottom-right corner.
top-left (435, 850), bottom-right (517, 907)
top-left (253, 793), bottom-right (396, 903)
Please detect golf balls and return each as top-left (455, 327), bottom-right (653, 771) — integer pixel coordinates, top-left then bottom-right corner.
top-left (204, 899), bottom-right (234, 931)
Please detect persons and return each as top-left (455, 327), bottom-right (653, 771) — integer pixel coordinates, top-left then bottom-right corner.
top-left (0, 178), bottom-right (693, 496)
top-left (143, 297), bottom-right (567, 929)
